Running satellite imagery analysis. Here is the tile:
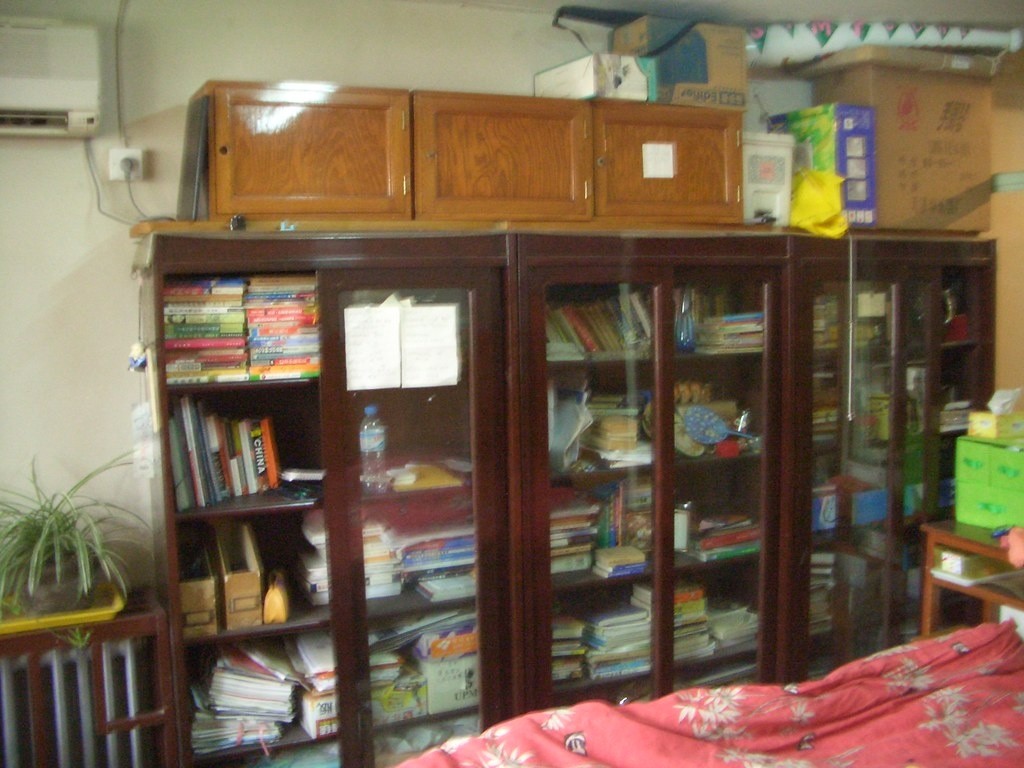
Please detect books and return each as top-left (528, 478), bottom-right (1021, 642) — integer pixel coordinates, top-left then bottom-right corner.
top-left (162, 272), bottom-right (927, 754)
top-left (930, 561), bottom-right (1024, 587)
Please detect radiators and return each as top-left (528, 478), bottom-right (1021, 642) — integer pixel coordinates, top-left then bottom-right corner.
top-left (0, 633), bottom-right (146, 768)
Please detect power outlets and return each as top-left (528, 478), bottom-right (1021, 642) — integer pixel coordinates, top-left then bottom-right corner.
top-left (109, 148), bottom-right (143, 181)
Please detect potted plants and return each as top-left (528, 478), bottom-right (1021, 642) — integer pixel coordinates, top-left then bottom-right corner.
top-left (0, 446), bottom-right (150, 618)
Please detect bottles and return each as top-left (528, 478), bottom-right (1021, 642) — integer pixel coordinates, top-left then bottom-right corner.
top-left (675, 279), bottom-right (698, 352)
top-left (359, 406), bottom-right (388, 495)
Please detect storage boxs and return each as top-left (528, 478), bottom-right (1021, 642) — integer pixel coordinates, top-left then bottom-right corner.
top-left (954, 435), bottom-right (1024, 530)
top-left (769, 99), bottom-right (878, 228)
top-left (536, 54), bottom-right (657, 103)
top-left (177, 525), bottom-right (216, 640)
top-left (215, 520), bottom-right (263, 630)
top-left (812, 66), bottom-right (992, 232)
top-left (611, 17), bottom-right (749, 111)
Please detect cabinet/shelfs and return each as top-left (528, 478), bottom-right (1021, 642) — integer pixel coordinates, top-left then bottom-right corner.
top-left (194, 79), bottom-right (743, 226)
top-left (156, 225), bottom-right (999, 768)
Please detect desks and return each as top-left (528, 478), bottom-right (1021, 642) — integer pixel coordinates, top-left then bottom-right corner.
top-left (918, 518), bottom-right (1024, 634)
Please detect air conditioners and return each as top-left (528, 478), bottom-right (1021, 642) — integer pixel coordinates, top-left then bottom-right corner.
top-left (0, 16), bottom-right (101, 140)
